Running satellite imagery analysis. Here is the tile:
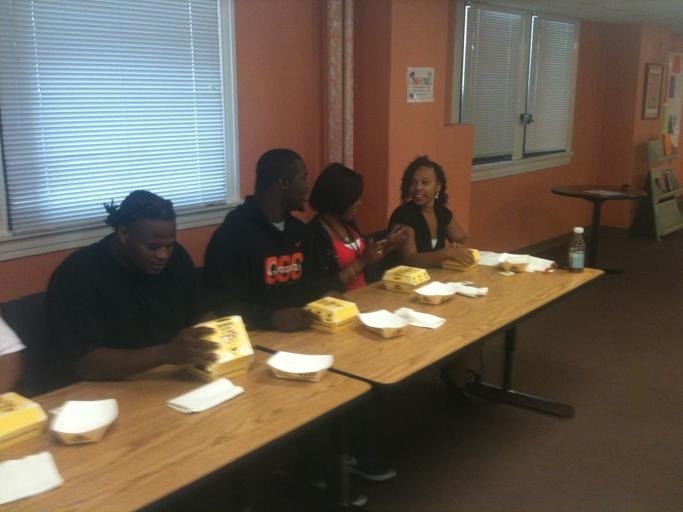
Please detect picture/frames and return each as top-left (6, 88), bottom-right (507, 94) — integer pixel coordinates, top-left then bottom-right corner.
top-left (641, 62), bottom-right (664, 120)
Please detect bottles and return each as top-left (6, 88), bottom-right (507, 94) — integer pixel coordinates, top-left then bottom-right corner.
top-left (569, 227), bottom-right (586, 273)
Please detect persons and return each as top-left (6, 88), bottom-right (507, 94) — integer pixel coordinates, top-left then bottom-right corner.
top-left (48, 189), bottom-right (219, 381)
top-left (0, 315), bottom-right (27, 397)
top-left (205, 149), bottom-right (407, 333)
top-left (388, 155), bottom-right (477, 406)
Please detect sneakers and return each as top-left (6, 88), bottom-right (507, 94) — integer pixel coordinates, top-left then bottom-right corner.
top-left (343, 458), bottom-right (397, 481)
top-left (338, 475), bottom-right (366, 507)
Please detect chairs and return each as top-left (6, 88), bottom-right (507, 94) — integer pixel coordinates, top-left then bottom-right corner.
top-left (8, 290), bottom-right (65, 391)
top-left (356, 230), bottom-right (408, 284)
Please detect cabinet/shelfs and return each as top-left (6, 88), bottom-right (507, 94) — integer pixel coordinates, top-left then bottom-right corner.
top-left (631, 139), bottom-right (682, 242)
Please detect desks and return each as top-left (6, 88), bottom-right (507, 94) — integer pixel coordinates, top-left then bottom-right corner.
top-left (550, 185), bottom-right (648, 275)
top-left (1, 347), bottom-right (374, 512)
top-left (247, 250), bottom-right (606, 425)
